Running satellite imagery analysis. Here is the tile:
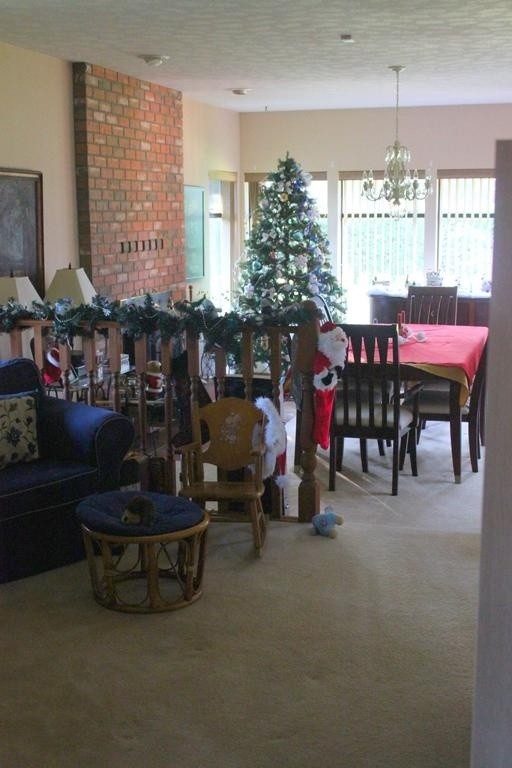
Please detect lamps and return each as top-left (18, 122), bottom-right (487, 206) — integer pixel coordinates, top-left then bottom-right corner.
top-left (359, 65), bottom-right (434, 207)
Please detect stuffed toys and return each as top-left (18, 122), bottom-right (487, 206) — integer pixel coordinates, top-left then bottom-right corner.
top-left (249, 394), bottom-right (289, 490)
top-left (285, 320), bottom-right (350, 451)
top-left (309, 504), bottom-right (343, 539)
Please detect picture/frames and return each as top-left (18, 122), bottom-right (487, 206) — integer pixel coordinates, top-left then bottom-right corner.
top-left (0, 166), bottom-right (45, 303)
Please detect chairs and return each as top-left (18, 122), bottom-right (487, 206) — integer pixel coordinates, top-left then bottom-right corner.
top-left (0, 358), bottom-right (134, 584)
top-left (173, 395), bottom-right (270, 562)
top-left (326, 284), bottom-right (489, 496)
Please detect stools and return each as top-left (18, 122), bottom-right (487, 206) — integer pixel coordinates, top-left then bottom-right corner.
top-left (75, 490), bottom-right (210, 614)
